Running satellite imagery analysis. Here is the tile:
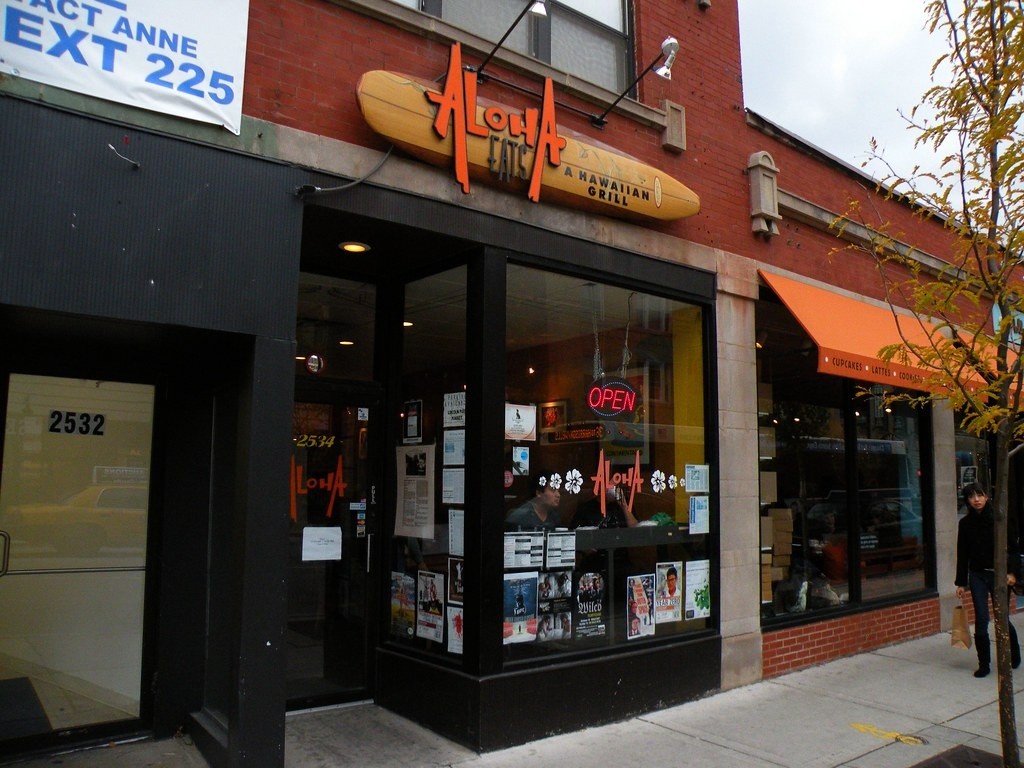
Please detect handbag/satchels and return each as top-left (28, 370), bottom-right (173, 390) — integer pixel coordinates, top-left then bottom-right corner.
top-left (950, 595), bottom-right (975, 650)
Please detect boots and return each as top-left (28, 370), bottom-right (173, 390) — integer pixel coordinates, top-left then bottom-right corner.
top-left (1008, 622), bottom-right (1021, 668)
top-left (973, 633), bottom-right (991, 677)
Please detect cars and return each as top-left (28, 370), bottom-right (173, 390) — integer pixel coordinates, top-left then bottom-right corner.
top-left (6, 466), bottom-right (149, 558)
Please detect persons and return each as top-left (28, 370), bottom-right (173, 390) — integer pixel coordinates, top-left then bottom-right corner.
top-left (570, 466), bottom-right (641, 607)
top-left (539, 567), bottom-right (681, 641)
top-left (816, 510), bottom-right (845, 543)
top-left (955, 483), bottom-right (1021, 678)
top-left (505, 472), bottom-right (562, 535)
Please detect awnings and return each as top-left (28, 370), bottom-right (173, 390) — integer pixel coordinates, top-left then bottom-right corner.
top-left (758, 267), bottom-right (1024, 410)
